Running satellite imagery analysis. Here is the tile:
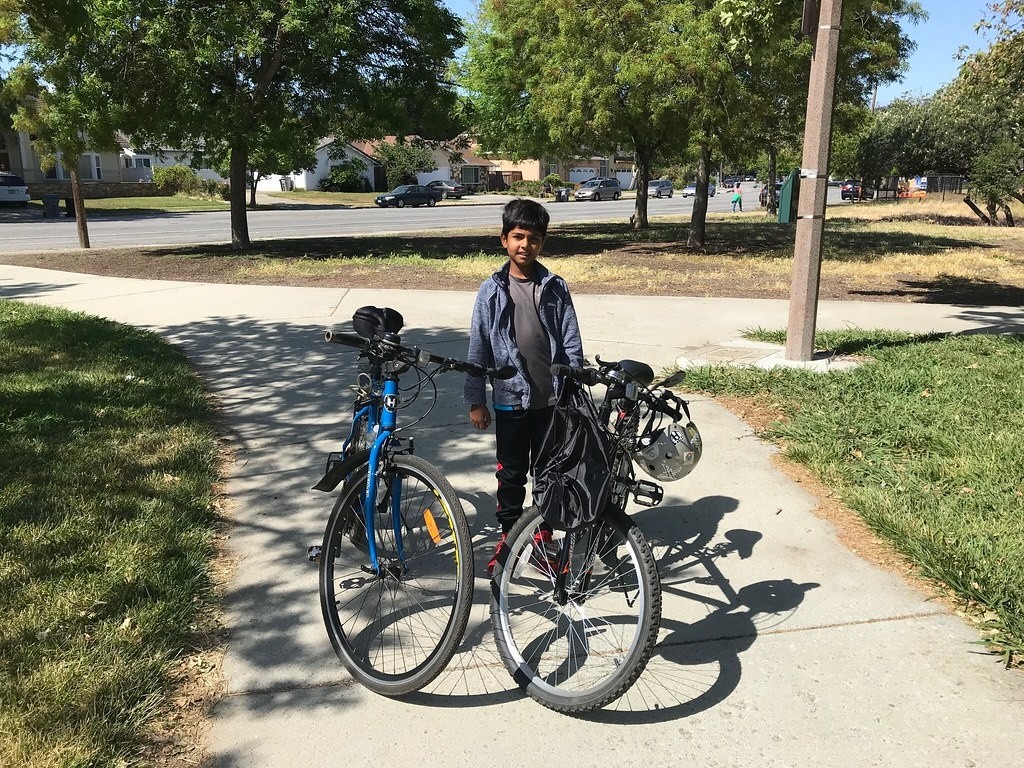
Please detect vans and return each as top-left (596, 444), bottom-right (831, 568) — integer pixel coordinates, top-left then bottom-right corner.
top-left (574, 180), bottom-right (621, 202)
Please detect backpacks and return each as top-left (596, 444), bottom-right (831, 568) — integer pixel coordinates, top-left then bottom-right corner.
top-left (533, 364), bottom-right (612, 530)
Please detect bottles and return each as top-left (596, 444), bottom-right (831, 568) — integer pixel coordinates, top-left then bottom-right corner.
top-left (365, 425), bottom-right (379, 449)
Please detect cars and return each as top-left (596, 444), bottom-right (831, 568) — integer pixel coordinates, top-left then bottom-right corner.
top-left (374, 185), bottom-right (442, 208)
top-left (827, 178), bottom-right (844, 188)
top-left (682, 182), bottom-right (716, 198)
top-left (0, 172), bottom-right (30, 208)
top-left (759, 183), bottom-right (784, 208)
top-left (723, 175), bottom-right (754, 188)
top-left (426, 180), bottom-right (468, 199)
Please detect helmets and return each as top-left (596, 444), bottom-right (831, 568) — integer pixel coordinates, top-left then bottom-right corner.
top-left (627, 422), bottom-right (703, 482)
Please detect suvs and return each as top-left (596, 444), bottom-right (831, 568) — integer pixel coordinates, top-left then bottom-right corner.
top-left (580, 176), bottom-right (618, 185)
top-left (647, 179), bottom-right (674, 198)
top-left (840, 179), bottom-right (874, 200)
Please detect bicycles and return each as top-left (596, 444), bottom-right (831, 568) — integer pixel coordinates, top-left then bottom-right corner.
top-left (489, 354), bottom-right (691, 717)
top-left (306, 304), bottom-right (518, 697)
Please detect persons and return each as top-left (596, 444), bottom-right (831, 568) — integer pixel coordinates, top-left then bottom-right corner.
top-left (897, 177), bottom-right (910, 197)
top-left (732, 183), bottom-right (743, 212)
top-left (465, 200), bottom-right (584, 575)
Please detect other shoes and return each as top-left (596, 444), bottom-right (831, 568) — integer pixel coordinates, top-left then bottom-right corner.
top-left (487, 531), bottom-right (507, 577)
top-left (533, 530), bottom-right (569, 575)
